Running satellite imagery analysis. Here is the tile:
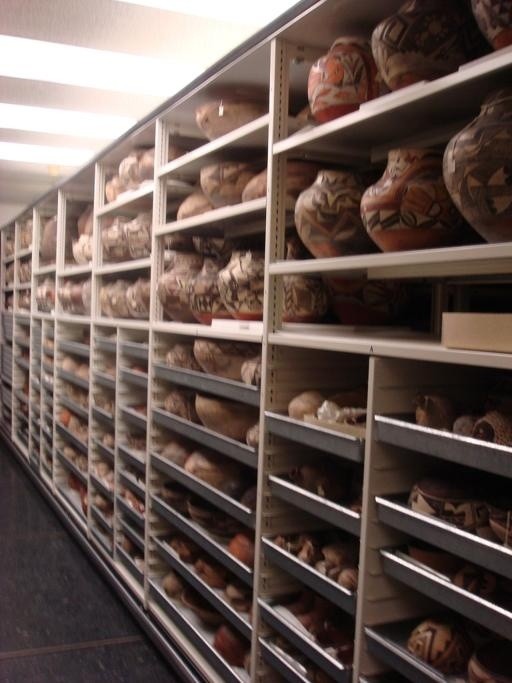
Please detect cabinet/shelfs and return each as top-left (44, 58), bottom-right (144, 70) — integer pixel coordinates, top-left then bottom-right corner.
top-left (0, 1), bottom-right (512, 682)
